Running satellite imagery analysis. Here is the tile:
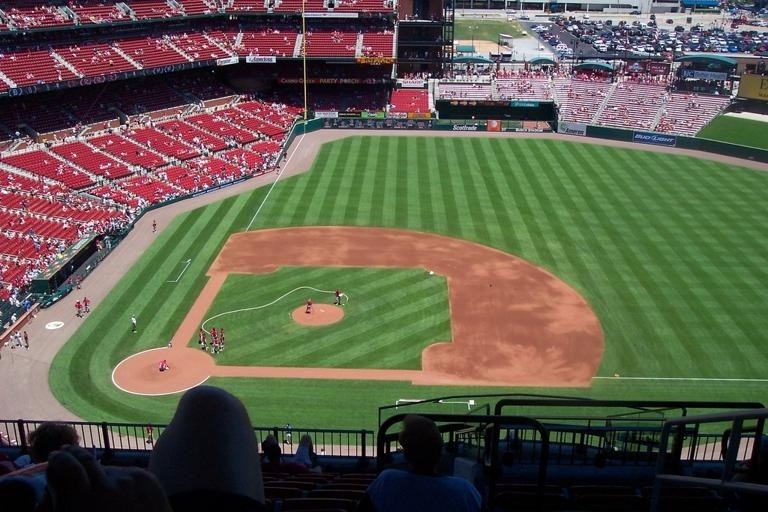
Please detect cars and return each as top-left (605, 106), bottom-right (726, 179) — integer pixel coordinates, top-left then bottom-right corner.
top-left (505, 2), bottom-right (767, 60)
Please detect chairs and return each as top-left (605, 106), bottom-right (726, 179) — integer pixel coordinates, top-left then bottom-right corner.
top-left (94, 456), bottom-right (732, 511)
top-left (1, 0), bottom-right (307, 336)
top-left (303, 0), bottom-right (398, 118)
top-left (389, 70), bottom-right (735, 139)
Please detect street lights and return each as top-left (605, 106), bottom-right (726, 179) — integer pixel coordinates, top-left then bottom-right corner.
top-left (468, 25), bottom-right (479, 57)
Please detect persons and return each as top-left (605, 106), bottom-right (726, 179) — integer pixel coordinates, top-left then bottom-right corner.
top-left (334, 289), bottom-right (342, 305)
top-left (159, 360), bottom-right (169, 372)
top-left (298, 1), bottom-right (768, 144)
top-left (305, 297), bottom-right (312, 314)
top-left (1, 385), bottom-right (766, 512)
top-left (0, 0), bottom-right (305, 355)
